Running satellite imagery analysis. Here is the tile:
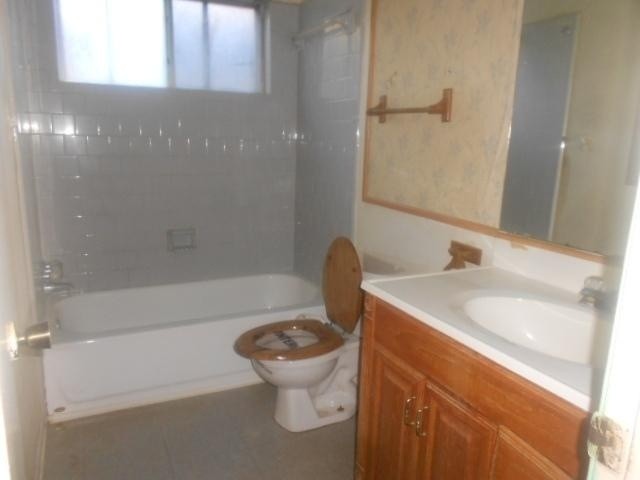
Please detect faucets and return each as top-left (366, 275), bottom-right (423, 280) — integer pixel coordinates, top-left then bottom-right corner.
top-left (38, 281), bottom-right (75, 298)
top-left (577, 277), bottom-right (614, 310)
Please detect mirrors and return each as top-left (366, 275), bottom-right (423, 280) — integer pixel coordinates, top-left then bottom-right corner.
top-left (497, 0), bottom-right (640, 263)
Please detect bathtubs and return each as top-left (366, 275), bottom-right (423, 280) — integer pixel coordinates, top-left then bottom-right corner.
top-left (39, 270), bottom-right (326, 426)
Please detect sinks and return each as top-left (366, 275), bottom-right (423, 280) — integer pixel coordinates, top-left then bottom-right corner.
top-left (460, 294), bottom-right (612, 414)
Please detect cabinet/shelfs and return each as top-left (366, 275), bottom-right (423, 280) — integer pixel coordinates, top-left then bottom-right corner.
top-left (357, 293), bottom-right (592, 479)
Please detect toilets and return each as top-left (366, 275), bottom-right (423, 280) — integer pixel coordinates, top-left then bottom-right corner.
top-left (233, 236), bottom-right (387, 433)
